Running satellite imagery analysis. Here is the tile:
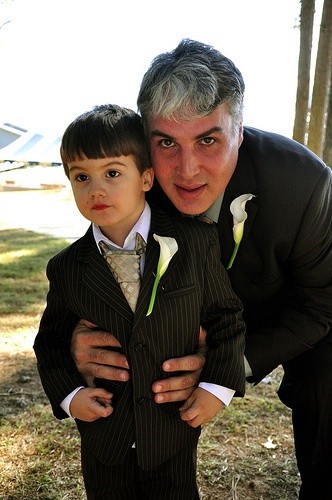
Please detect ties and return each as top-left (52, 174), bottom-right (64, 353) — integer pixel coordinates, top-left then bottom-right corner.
top-left (101, 232), bottom-right (147, 318)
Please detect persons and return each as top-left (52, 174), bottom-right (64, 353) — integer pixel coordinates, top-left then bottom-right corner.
top-left (67, 38), bottom-right (332, 499)
top-left (33, 103), bottom-right (248, 500)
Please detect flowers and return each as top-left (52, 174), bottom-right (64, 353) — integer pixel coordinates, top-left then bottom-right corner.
top-left (226, 194), bottom-right (257, 269)
top-left (145, 232), bottom-right (179, 317)
